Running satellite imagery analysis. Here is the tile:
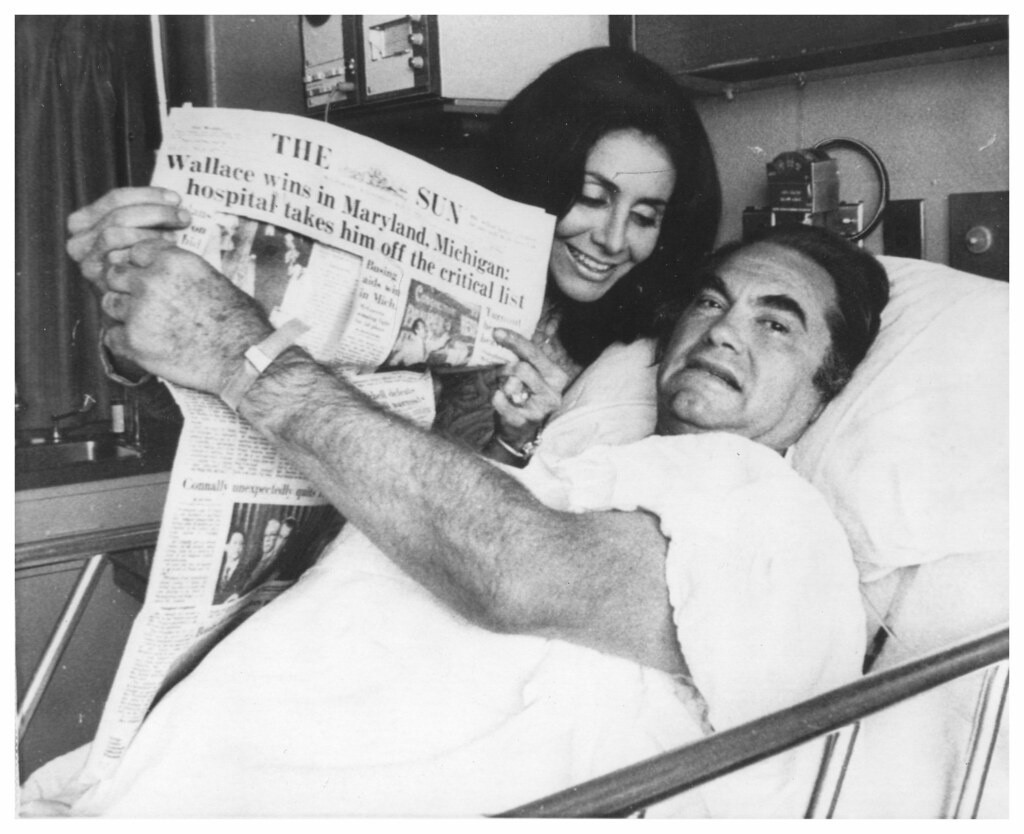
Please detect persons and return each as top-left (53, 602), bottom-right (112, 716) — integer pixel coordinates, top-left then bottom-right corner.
top-left (64, 46), bottom-right (722, 470)
top-left (389, 318), bottom-right (430, 371)
top-left (215, 516), bottom-right (296, 604)
top-left (15, 217), bottom-right (889, 819)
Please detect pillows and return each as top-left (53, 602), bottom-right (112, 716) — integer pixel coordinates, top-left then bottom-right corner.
top-left (534, 252), bottom-right (1011, 586)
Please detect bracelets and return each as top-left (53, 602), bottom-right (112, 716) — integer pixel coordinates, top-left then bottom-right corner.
top-left (221, 319), bottom-right (312, 414)
top-left (496, 432), bottom-right (540, 459)
top-left (97, 326), bottom-right (154, 387)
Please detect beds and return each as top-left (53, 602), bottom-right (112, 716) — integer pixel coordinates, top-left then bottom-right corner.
top-left (15, 522), bottom-right (1010, 817)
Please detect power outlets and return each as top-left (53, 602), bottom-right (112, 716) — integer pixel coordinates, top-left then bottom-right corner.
top-left (883, 200), bottom-right (922, 259)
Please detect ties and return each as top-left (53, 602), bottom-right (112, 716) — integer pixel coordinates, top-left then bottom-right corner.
top-left (422, 340), bottom-right (427, 360)
top-left (223, 566), bottom-right (232, 583)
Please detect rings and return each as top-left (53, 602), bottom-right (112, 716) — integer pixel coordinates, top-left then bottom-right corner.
top-left (511, 390), bottom-right (529, 406)
